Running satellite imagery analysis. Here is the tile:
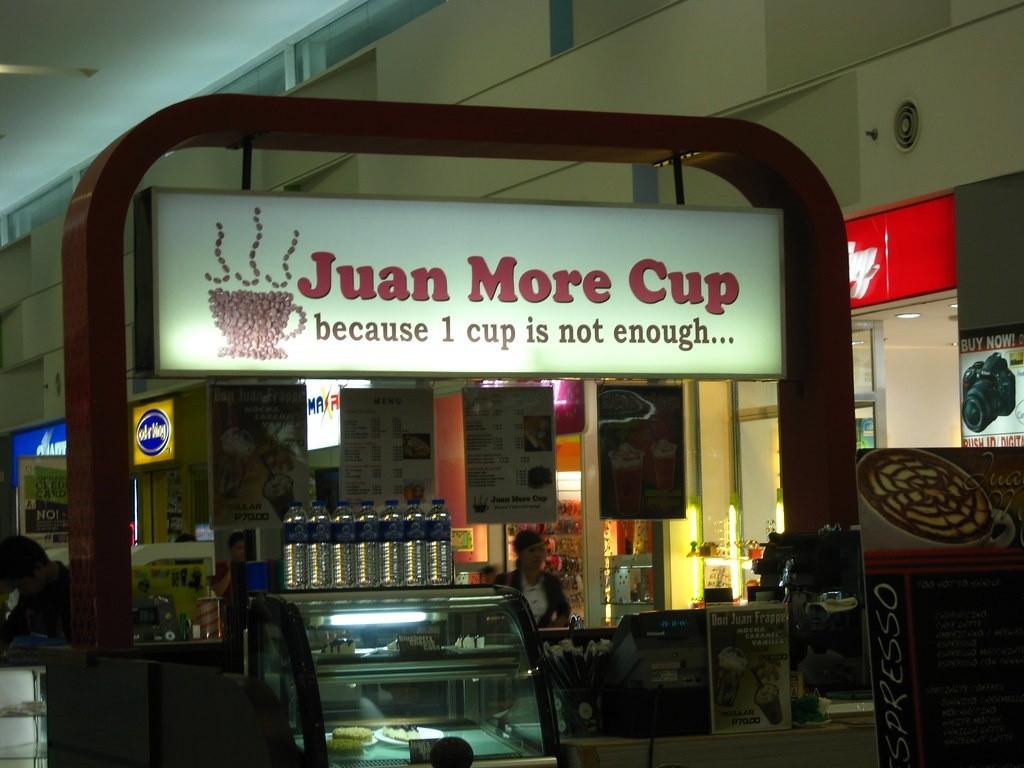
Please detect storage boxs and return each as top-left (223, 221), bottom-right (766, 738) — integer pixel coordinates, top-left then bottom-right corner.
top-left (245, 560), bottom-right (267, 590)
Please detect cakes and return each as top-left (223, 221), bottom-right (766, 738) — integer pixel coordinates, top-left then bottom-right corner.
top-left (327, 726), bottom-right (373, 757)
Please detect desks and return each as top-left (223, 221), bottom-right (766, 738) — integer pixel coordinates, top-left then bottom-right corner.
top-left (510, 715), bottom-right (878, 768)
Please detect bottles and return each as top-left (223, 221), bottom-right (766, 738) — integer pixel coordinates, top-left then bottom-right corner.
top-left (354, 500), bottom-right (381, 587)
top-left (378, 500), bottom-right (404, 587)
top-left (425, 499), bottom-right (453, 586)
top-left (305, 501), bottom-right (332, 589)
top-left (177, 613), bottom-right (191, 641)
top-left (401, 499), bottom-right (426, 586)
top-left (331, 500), bottom-right (357, 588)
top-left (283, 501), bottom-right (308, 589)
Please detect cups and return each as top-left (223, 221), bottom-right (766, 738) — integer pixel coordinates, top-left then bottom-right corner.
top-left (262, 473), bottom-right (295, 518)
top-left (856, 447), bottom-right (1015, 550)
top-left (651, 444), bottom-right (677, 488)
top-left (754, 683), bottom-right (783, 725)
top-left (215, 426), bottom-right (255, 499)
top-left (714, 646), bottom-right (747, 706)
top-left (608, 449), bottom-right (646, 516)
top-left (551, 688), bottom-right (603, 738)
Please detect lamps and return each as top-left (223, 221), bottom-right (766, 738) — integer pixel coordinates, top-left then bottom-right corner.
top-left (309, 612), bottom-right (448, 627)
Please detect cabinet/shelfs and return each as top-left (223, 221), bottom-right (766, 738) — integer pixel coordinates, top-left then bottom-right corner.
top-left (246, 583), bottom-right (564, 768)
top-left (41, 540), bottom-right (216, 640)
top-left (0, 660), bottom-right (50, 768)
top-left (609, 554), bottom-right (653, 625)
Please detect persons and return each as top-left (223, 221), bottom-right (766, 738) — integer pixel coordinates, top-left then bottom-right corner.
top-left (478, 566), bottom-right (496, 583)
top-left (223, 532), bottom-right (255, 621)
top-left (0, 535), bottom-right (71, 646)
top-left (494, 530), bottom-right (572, 629)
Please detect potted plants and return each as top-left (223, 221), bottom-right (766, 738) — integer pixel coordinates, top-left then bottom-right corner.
top-left (700, 542), bottom-right (717, 556)
top-left (692, 597), bottom-right (704, 609)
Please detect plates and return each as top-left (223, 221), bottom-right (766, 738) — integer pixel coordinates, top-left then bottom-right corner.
top-left (312, 647), bottom-right (377, 654)
top-left (827, 702), bottom-right (875, 718)
top-left (375, 646), bottom-right (445, 652)
top-left (443, 644), bottom-right (514, 654)
top-left (325, 731), bottom-right (378, 747)
top-left (792, 719), bottom-right (832, 728)
top-left (374, 727), bottom-right (444, 746)
